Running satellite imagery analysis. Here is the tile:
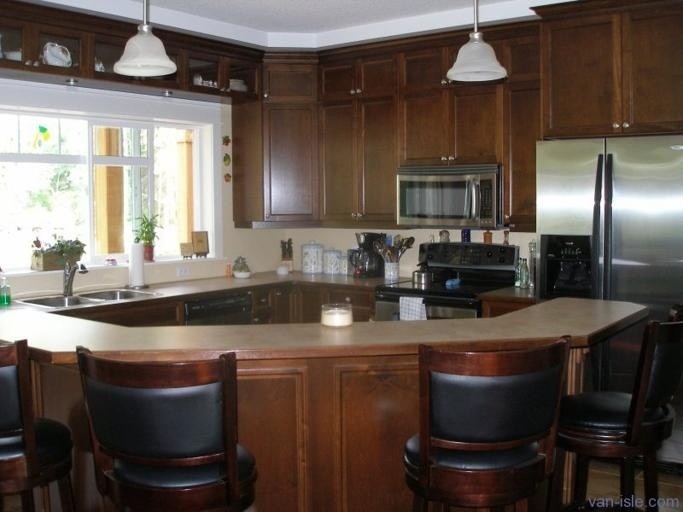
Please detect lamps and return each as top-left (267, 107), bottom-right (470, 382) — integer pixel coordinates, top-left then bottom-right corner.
top-left (446, 0), bottom-right (507, 82)
top-left (114, 0), bottom-right (177, 78)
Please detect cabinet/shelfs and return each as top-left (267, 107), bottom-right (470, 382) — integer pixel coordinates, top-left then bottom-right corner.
top-left (293, 287), bottom-right (374, 321)
top-left (541, 0), bottom-right (683, 139)
top-left (232, 19), bottom-right (541, 233)
top-left (0, 14), bottom-right (261, 103)
top-left (253, 287), bottom-right (293, 325)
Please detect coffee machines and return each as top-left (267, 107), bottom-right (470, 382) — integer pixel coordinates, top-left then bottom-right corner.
top-left (349, 231), bottom-right (387, 278)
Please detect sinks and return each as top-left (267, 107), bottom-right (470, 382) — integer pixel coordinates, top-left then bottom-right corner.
top-left (22, 296), bottom-right (103, 315)
top-left (79, 290), bottom-right (154, 308)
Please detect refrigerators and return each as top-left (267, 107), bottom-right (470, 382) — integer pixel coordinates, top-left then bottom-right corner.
top-left (535, 134), bottom-right (683, 465)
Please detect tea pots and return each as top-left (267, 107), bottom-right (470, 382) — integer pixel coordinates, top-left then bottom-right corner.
top-left (411, 261), bottom-right (435, 285)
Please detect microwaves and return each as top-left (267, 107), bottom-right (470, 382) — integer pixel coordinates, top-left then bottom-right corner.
top-left (396, 163), bottom-right (505, 230)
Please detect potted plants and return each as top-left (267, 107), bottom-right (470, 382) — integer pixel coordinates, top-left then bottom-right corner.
top-left (137, 215), bottom-right (162, 262)
top-left (33, 240), bottom-right (85, 270)
top-left (233, 256), bottom-right (251, 279)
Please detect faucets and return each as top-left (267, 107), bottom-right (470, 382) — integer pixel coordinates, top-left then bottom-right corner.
top-left (66, 265), bottom-right (80, 296)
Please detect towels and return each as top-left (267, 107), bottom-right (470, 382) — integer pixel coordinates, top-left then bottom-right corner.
top-left (399, 296), bottom-right (427, 320)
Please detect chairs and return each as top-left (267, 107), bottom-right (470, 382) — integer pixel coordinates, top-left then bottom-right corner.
top-left (557, 305), bottom-right (683, 512)
top-left (0, 339), bottom-right (74, 512)
top-left (402, 333), bottom-right (570, 512)
top-left (75, 346), bottom-right (258, 512)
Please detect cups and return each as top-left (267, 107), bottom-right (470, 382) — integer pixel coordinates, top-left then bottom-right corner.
top-left (384, 263), bottom-right (399, 279)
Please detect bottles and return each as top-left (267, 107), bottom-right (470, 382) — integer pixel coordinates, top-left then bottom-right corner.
top-left (515, 258), bottom-right (529, 288)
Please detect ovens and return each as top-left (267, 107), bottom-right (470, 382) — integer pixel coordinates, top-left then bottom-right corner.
top-left (376, 301), bottom-right (482, 321)
